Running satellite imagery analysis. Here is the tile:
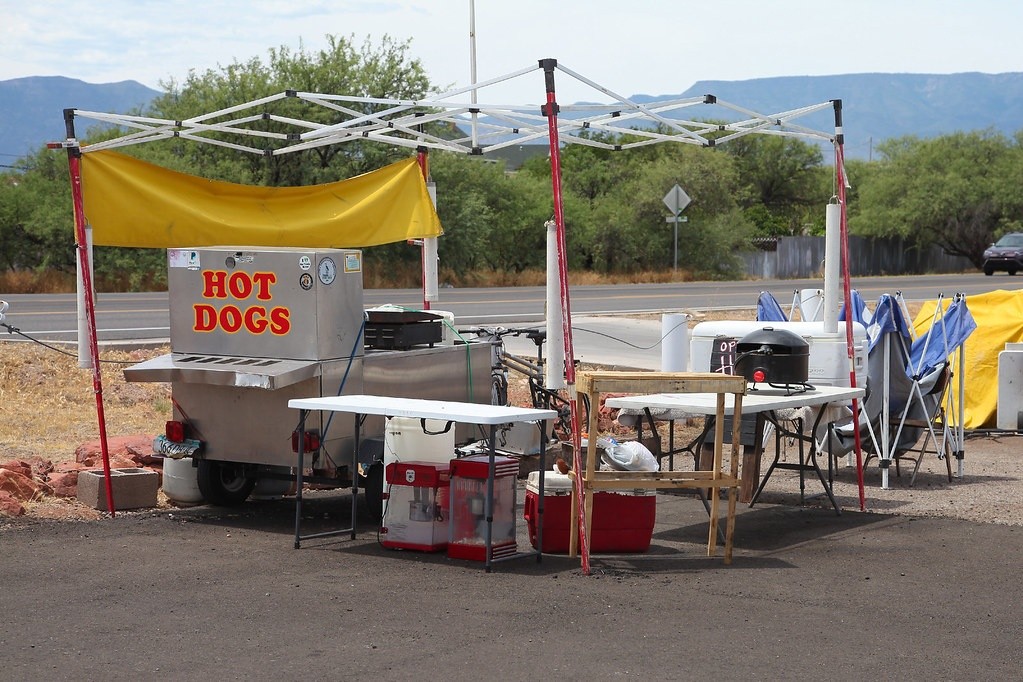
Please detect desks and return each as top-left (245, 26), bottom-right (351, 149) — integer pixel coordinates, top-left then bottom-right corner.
top-left (287, 395), bottom-right (557, 573)
top-left (568, 369), bottom-right (747, 566)
top-left (605, 383), bottom-right (866, 544)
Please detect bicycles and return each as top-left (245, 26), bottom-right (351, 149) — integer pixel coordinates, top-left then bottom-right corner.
top-left (457, 327), bottom-right (590, 440)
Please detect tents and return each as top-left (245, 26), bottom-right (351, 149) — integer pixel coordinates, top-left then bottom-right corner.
top-left (63, 59), bottom-right (867, 519)
top-left (758, 291), bottom-right (977, 490)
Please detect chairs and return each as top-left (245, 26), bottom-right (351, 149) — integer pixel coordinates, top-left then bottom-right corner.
top-left (863, 367), bottom-right (952, 487)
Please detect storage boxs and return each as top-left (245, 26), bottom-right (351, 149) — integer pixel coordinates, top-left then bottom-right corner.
top-left (523, 471), bottom-right (656, 553)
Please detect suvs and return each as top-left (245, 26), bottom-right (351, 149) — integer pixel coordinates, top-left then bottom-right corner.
top-left (982, 231), bottom-right (1023, 276)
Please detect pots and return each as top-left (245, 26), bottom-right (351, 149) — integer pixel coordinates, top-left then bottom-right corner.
top-left (734, 325), bottom-right (821, 396)
